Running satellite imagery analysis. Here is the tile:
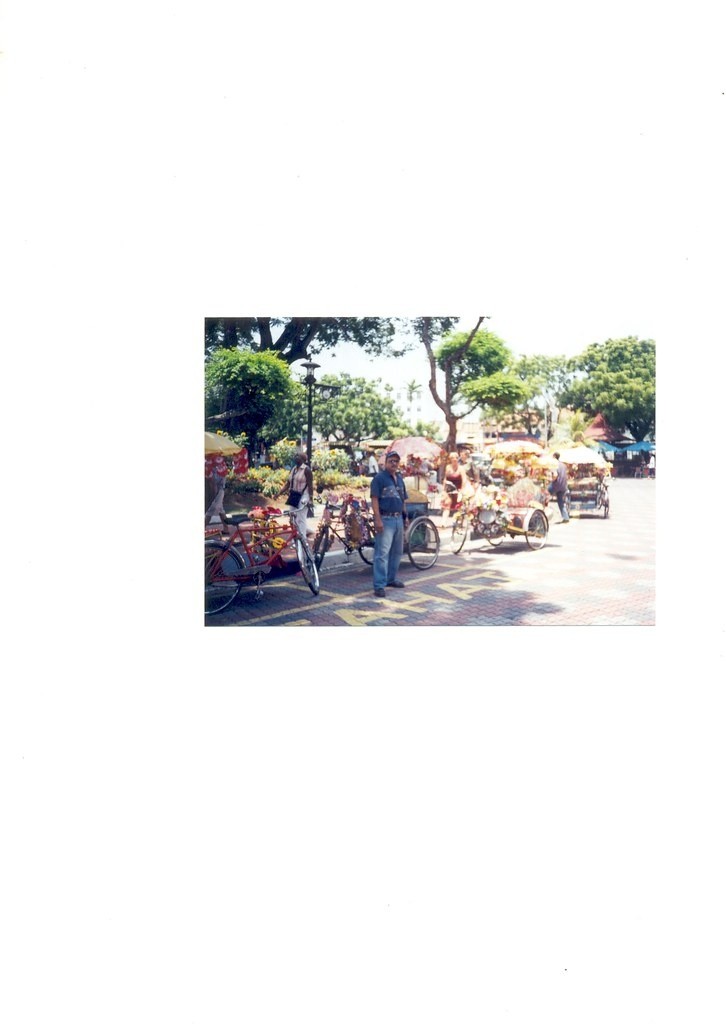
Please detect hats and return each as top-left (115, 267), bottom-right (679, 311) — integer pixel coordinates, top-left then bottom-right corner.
top-left (387, 451), bottom-right (400, 460)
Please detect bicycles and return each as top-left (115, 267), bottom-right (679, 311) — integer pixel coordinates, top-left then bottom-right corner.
top-left (206, 499), bottom-right (320, 617)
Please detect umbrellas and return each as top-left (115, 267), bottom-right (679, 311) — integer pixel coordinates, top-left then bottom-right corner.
top-left (384, 436), bottom-right (446, 475)
top-left (534, 440), bottom-right (608, 480)
top-left (484, 439), bottom-right (544, 478)
top-left (205, 431), bottom-right (241, 456)
top-left (582, 412), bottom-right (656, 477)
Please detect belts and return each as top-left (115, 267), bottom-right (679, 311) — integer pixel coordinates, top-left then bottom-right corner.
top-left (379, 510), bottom-right (402, 517)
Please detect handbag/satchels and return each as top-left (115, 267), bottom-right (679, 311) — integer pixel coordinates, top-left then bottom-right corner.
top-left (285, 491), bottom-right (302, 509)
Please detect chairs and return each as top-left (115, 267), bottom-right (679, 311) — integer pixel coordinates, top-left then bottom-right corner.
top-left (507, 478), bottom-right (541, 519)
top-left (567, 476), bottom-right (598, 491)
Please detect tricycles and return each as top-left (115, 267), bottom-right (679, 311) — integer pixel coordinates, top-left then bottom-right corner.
top-left (313, 494), bottom-right (440, 570)
top-left (445, 479), bottom-right (550, 555)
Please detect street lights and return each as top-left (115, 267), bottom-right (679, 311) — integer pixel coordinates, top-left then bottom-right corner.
top-left (300, 357), bottom-right (321, 518)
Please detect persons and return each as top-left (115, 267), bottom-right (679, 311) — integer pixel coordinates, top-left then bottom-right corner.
top-left (647, 453), bottom-right (655, 478)
top-left (547, 452), bottom-right (569, 524)
top-left (358, 452), bottom-right (438, 478)
top-left (273, 451), bottom-right (313, 550)
top-left (205, 476), bottom-right (230, 535)
top-left (442, 446), bottom-right (480, 535)
top-left (370, 451), bottom-right (409, 598)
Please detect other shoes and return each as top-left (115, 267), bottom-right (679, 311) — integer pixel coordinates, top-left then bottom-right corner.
top-left (375, 588), bottom-right (385, 597)
top-left (387, 581), bottom-right (404, 588)
